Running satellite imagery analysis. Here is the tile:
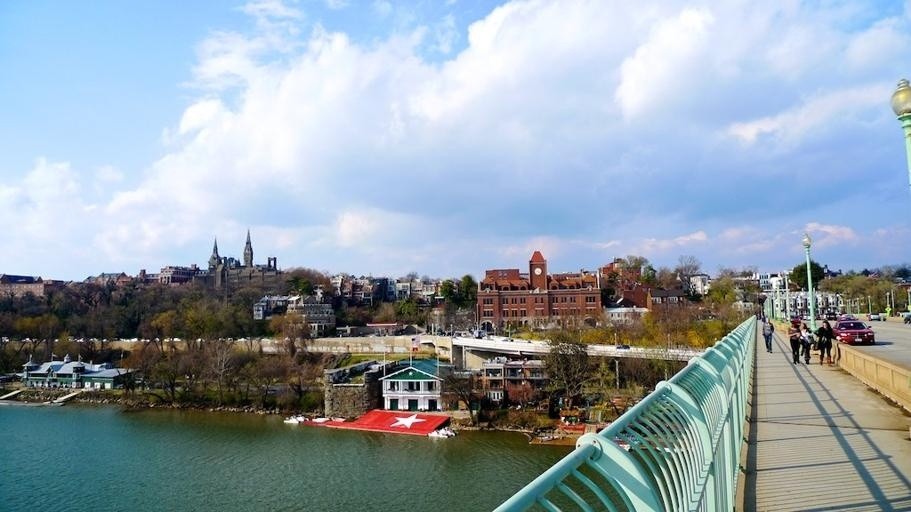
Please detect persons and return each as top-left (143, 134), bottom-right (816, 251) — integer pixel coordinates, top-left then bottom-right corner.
top-left (814, 321), bottom-right (841, 365)
top-left (788, 320), bottom-right (813, 364)
top-left (762, 317), bottom-right (774, 353)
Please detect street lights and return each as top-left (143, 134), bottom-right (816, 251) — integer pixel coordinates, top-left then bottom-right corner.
top-left (802, 232), bottom-right (818, 333)
top-left (906, 288), bottom-right (911, 306)
top-left (885, 292), bottom-right (889, 305)
top-left (614, 332), bottom-right (616, 346)
top-left (846, 295), bottom-right (872, 314)
top-left (667, 333), bottom-right (671, 349)
top-left (607, 357), bottom-right (622, 391)
top-left (508, 323), bottom-right (510, 338)
top-left (449, 323), bottom-right (452, 337)
top-left (414, 323), bottom-right (417, 335)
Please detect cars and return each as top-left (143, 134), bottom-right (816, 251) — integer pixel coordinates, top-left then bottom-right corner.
top-left (366, 328), bottom-right (514, 343)
top-left (785, 310), bottom-right (859, 323)
top-left (763, 270), bottom-right (791, 323)
top-left (868, 312), bottom-right (881, 320)
top-left (333, 330), bottom-right (352, 337)
top-left (830, 318), bottom-right (875, 346)
top-left (1, 336), bottom-right (291, 344)
top-left (572, 343), bottom-right (586, 351)
top-left (904, 314), bottom-right (911, 324)
top-left (615, 345), bottom-right (630, 350)
top-left (578, 328), bottom-right (582, 344)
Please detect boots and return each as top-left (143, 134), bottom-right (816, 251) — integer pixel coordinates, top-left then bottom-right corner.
top-left (792, 355), bottom-right (833, 364)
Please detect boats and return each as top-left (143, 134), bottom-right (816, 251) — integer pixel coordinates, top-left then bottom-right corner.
top-left (426, 426), bottom-right (459, 439)
top-left (282, 415), bottom-right (309, 424)
